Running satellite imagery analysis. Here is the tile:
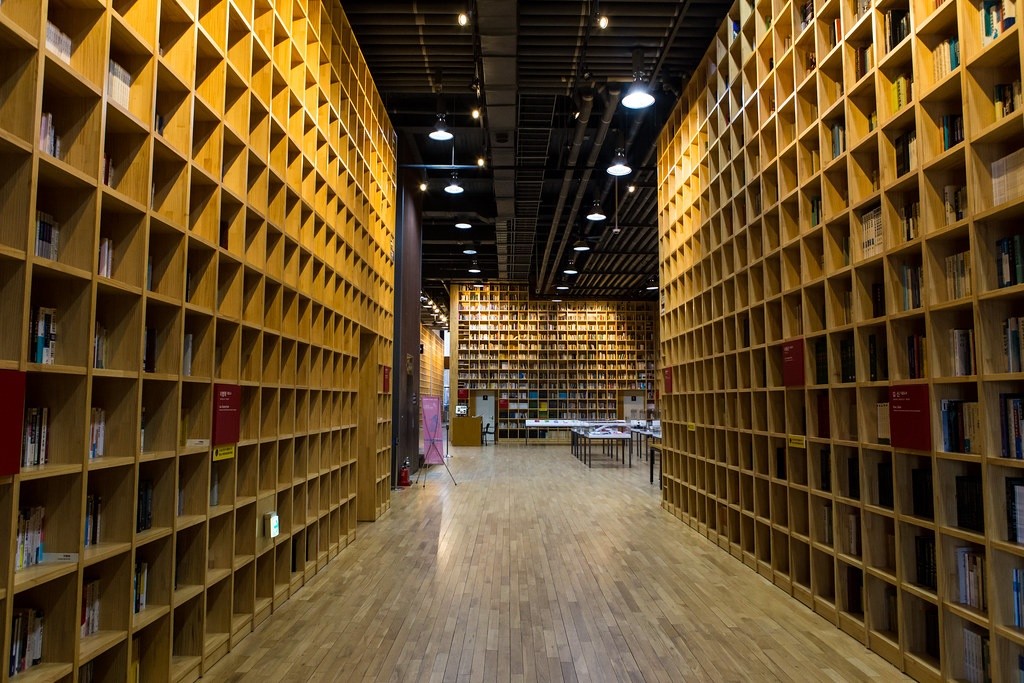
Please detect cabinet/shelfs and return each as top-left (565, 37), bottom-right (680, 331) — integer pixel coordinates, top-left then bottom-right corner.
top-left (657, 0), bottom-right (1024, 683)
top-left (449, 283), bottom-right (660, 444)
top-left (0, 0), bottom-right (443, 683)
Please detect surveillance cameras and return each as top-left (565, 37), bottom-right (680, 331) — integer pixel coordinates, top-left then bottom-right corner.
top-left (613, 229), bottom-right (620, 234)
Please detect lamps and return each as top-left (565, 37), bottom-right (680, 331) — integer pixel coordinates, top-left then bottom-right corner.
top-left (607, 133), bottom-right (632, 177)
top-left (466, 264), bottom-right (484, 273)
top-left (463, 247), bottom-right (477, 255)
top-left (457, 10), bottom-right (473, 27)
top-left (552, 291), bottom-right (562, 302)
top-left (587, 188), bottom-right (606, 221)
top-left (564, 252), bottom-right (578, 274)
top-left (420, 179), bottom-right (430, 192)
top-left (455, 216), bottom-right (472, 229)
top-left (567, 107), bottom-right (580, 120)
top-left (557, 275), bottom-right (569, 290)
top-left (444, 169), bottom-right (464, 194)
top-left (622, 48), bottom-right (654, 110)
top-left (592, 12), bottom-right (608, 29)
top-left (428, 97), bottom-right (457, 141)
top-left (573, 227), bottom-right (590, 251)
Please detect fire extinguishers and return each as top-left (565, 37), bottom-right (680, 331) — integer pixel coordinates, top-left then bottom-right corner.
top-left (400, 457), bottom-right (410, 486)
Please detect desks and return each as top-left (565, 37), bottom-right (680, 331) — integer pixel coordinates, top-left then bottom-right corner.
top-left (525, 420), bottom-right (662, 490)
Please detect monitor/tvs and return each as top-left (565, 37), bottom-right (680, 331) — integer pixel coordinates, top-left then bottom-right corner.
top-left (455, 405), bottom-right (467, 416)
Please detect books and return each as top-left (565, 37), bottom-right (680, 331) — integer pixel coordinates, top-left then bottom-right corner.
top-left (733, 0), bottom-right (1024, 683)
top-left (9, 21), bottom-right (220, 683)
top-left (458, 285), bottom-right (661, 438)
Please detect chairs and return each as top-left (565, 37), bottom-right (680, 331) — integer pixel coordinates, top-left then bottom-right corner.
top-left (484, 423), bottom-right (498, 444)
top-left (482, 424), bottom-right (490, 445)
